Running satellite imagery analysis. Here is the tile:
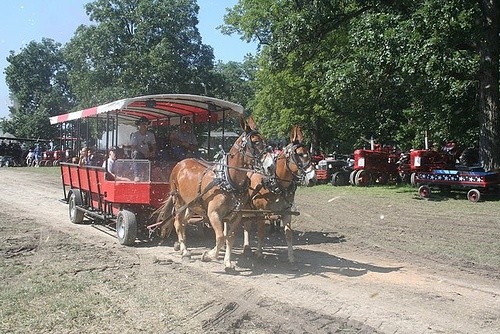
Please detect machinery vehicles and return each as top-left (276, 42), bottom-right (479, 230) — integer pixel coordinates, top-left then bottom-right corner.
top-left (410, 130), bottom-right (466, 188)
top-left (350, 135), bottom-right (410, 185)
top-left (315, 157), bottom-right (355, 186)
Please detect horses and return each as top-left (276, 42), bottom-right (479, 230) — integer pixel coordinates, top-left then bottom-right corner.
top-left (148, 115), bottom-right (318, 272)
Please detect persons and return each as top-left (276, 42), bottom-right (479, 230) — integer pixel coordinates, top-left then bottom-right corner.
top-left (26, 150), bottom-right (34, 166)
top-left (78, 148), bottom-right (117, 178)
top-left (131, 116), bottom-right (157, 181)
top-left (169, 121), bottom-right (200, 162)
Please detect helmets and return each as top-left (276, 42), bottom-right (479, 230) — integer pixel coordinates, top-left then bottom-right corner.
top-left (134, 116), bottom-right (151, 125)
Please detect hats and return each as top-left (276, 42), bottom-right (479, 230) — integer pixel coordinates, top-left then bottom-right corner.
top-left (108, 146), bottom-right (120, 151)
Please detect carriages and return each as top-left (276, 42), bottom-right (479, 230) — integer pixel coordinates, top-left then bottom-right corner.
top-left (49, 93), bottom-right (316, 276)
